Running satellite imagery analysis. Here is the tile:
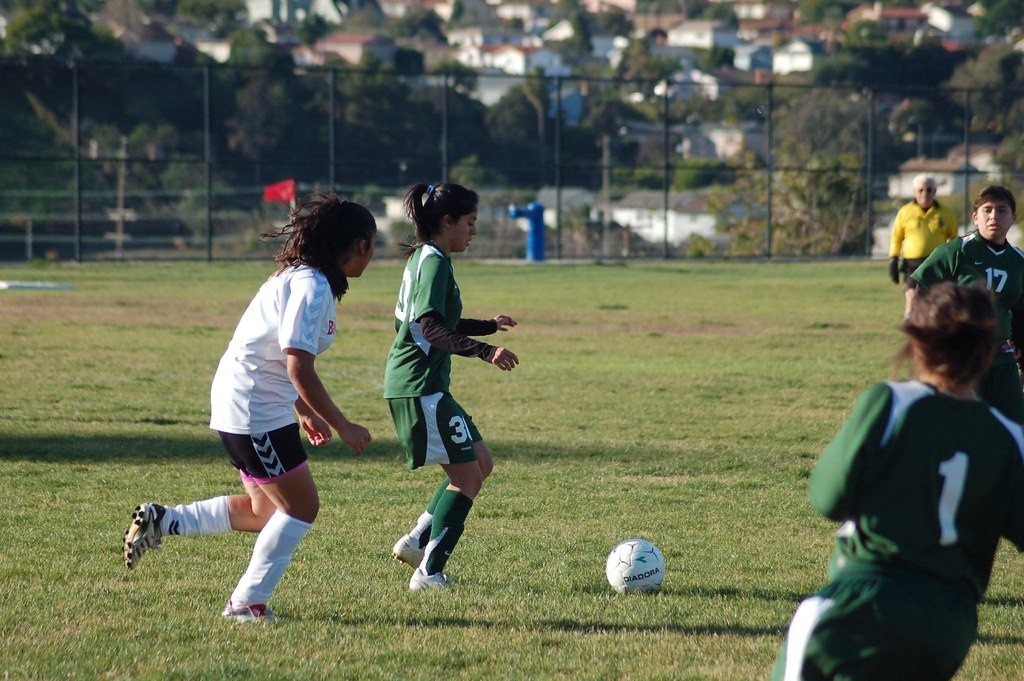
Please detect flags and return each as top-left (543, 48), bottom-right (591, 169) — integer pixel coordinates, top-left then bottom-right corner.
top-left (263, 178), bottom-right (296, 202)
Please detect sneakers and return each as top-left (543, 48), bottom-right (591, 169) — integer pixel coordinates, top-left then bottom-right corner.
top-left (408, 566), bottom-right (454, 593)
top-left (390, 533), bottom-right (425, 569)
top-left (220, 599), bottom-right (281, 622)
top-left (121, 504), bottom-right (167, 569)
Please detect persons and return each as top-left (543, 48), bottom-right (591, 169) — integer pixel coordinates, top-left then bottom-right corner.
top-left (888, 169), bottom-right (959, 290)
top-left (769, 279), bottom-right (1024, 681)
top-left (122, 192), bottom-right (378, 623)
top-left (907, 184), bottom-right (1024, 424)
top-left (383, 181), bottom-right (519, 592)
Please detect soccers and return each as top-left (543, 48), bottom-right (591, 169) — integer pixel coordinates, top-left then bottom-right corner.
top-left (605, 537), bottom-right (667, 595)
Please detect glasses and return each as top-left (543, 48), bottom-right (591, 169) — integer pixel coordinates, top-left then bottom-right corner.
top-left (917, 188), bottom-right (934, 194)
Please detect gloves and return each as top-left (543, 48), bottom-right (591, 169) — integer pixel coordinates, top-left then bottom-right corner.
top-left (889, 257), bottom-right (900, 284)
